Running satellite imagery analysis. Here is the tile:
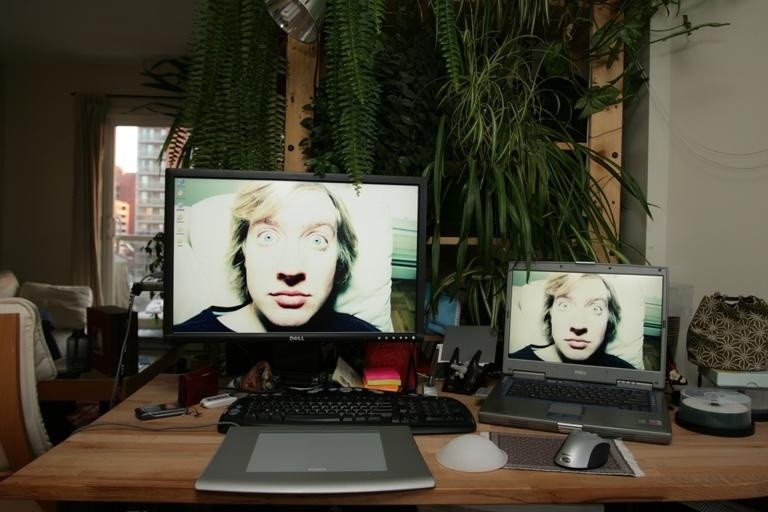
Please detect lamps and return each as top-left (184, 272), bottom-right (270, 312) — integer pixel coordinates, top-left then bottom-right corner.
top-left (0, 298), bottom-right (60, 512)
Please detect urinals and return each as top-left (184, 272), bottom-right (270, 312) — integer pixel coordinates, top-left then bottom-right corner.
top-left (362, 367), bottom-right (401, 393)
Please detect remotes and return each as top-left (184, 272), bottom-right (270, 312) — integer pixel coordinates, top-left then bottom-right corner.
top-left (553, 430), bottom-right (611, 470)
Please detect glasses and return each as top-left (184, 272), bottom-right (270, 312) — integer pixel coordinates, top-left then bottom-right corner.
top-left (264, 0), bottom-right (327, 44)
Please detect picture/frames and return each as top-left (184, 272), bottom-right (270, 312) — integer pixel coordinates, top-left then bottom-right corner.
top-left (1, 373), bottom-right (768, 506)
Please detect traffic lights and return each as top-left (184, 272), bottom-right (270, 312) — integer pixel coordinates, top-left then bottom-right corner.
top-left (1, 271), bottom-right (94, 381)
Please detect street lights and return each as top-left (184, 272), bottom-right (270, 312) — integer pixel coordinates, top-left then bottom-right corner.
top-left (686, 292), bottom-right (768, 371)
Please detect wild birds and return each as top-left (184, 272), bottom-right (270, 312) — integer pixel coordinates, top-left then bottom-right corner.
top-left (194, 426), bottom-right (437, 495)
top-left (477, 260), bottom-right (673, 446)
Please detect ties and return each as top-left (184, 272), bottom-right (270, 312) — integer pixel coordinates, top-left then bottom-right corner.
top-left (163, 168), bottom-right (427, 347)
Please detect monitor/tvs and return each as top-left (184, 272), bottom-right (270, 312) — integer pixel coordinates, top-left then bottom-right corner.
top-left (331, 356), bottom-right (403, 393)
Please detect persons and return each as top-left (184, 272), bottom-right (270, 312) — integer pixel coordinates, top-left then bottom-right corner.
top-left (509, 273), bottom-right (637, 369)
top-left (172, 181), bottom-right (380, 333)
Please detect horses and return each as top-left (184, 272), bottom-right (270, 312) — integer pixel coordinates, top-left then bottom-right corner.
top-left (134, 402), bottom-right (187, 421)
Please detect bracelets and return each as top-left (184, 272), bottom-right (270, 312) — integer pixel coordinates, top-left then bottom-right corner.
top-left (437, 435), bottom-right (507, 472)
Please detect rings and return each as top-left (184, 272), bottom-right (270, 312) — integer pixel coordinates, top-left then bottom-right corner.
top-left (427, 343), bottom-right (444, 387)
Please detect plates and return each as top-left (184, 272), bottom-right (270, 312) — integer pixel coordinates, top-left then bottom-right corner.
top-left (285, 1), bottom-right (625, 344)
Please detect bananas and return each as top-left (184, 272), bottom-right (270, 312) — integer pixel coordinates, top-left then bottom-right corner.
top-left (216, 394), bottom-right (477, 435)
